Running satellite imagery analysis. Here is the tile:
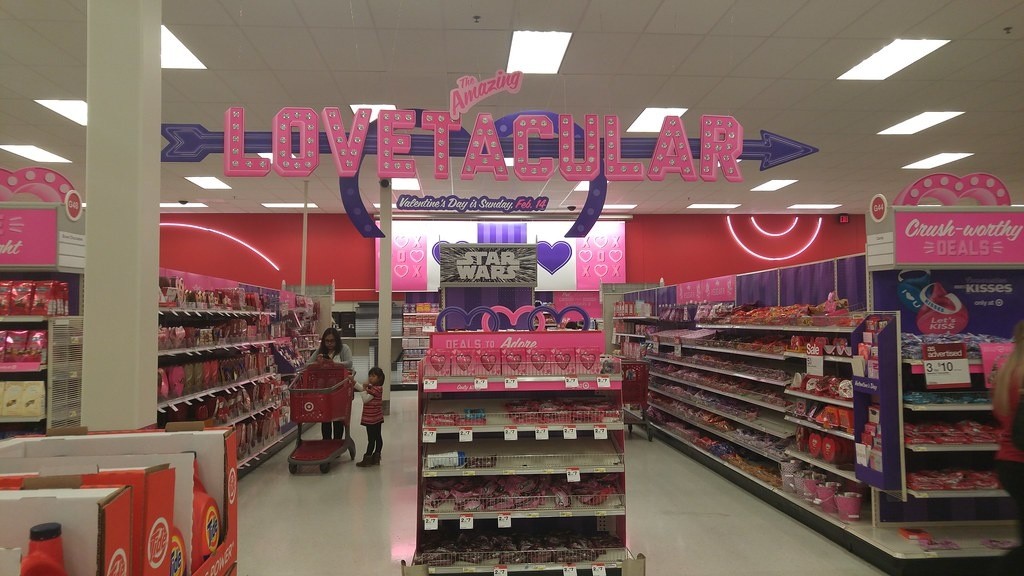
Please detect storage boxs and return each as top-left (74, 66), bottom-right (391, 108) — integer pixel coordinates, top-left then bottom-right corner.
top-left (332, 301), bottom-right (438, 384)
top-left (0, 421), bottom-right (238, 576)
top-left (0, 280), bottom-right (69, 417)
top-left (158, 273), bottom-right (293, 460)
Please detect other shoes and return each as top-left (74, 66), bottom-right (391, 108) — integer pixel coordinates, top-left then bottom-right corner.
top-left (371, 452), bottom-right (381, 464)
top-left (356, 454), bottom-right (372, 467)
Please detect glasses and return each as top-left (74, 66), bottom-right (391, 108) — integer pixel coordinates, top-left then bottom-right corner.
top-left (325, 340), bottom-right (336, 343)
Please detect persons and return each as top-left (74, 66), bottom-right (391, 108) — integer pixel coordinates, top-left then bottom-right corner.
top-left (983, 320), bottom-right (1024, 576)
top-left (303, 328), bottom-right (354, 457)
top-left (347, 367), bottom-right (385, 466)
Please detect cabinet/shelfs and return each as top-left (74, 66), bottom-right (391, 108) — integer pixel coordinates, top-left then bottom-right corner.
top-left (614, 288), bottom-right (1014, 523)
top-left (279, 327), bottom-right (322, 378)
top-left (403, 313), bottom-right (629, 574)
top-left (158, 305), bottom-right (284, 474)
top-left (0, 267), bottom-right (84, 422)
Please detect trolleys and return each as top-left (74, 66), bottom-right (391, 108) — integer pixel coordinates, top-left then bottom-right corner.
top-left (287, 354), bottom-right (356, 474)
top-left (616, 357), bottom-right (654, 442)
